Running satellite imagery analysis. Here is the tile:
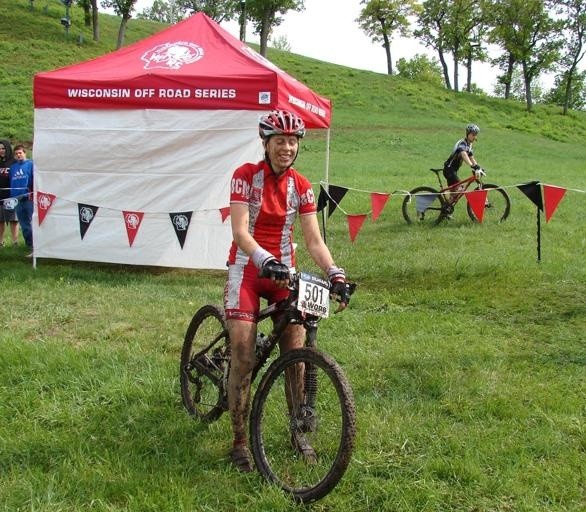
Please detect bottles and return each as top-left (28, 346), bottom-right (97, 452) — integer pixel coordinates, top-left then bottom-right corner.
top-left (254, 332), bottom-right (266, 348)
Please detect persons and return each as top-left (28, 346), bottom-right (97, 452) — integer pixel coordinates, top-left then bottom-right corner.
top-left (223, 110), bottom-right (351, 474)
top-left (440, 124), bottom-right (482, 221)
top-left (8, 145), bottom-right (33, 257)
top-left (0, 140), bottom-right (19, 248)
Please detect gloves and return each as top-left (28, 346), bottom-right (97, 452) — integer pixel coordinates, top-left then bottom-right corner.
top-left (475, 167), bottom-right (486, 179)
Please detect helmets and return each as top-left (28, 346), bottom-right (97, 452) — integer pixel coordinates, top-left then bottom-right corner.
top-left (466, 124), bottom-right (479, 133)
top-left (259, 110), bottom-right (306, 139)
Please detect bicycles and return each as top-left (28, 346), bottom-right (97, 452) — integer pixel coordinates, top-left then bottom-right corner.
top-left (401, 164), bottom-right (513, 229)
top-left (179, 266), bottom-right (357, 502)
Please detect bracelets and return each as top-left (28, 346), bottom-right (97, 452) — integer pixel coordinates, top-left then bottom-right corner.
top-left (326, 265), bottom-right (346, 282)
top-left (252, 247), bottom-right (277, 269)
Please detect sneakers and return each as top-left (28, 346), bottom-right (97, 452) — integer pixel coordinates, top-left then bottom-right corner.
top-left (294, 441), bottom-right (317, 462)
top-left (232, 445), bottom-right (251, 472)
